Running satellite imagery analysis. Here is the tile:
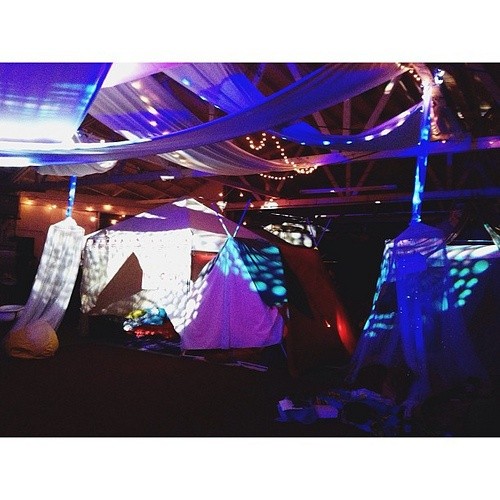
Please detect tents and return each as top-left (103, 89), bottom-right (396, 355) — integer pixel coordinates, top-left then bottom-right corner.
top-left (77, 199), bottom-right (344, 380)
